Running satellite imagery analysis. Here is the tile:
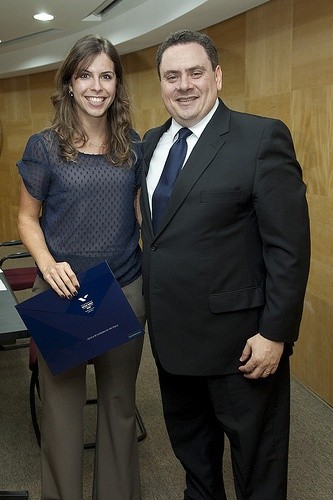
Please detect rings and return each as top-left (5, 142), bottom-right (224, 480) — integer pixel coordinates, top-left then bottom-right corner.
top-left (264, 371), bottom-right (271, 376)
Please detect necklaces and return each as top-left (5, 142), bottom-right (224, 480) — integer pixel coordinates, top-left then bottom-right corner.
top-left (81, 138), bottom-right (102, 148)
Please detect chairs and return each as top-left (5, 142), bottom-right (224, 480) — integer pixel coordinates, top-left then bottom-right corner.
top-left (0, 241), bottom-right (147, 456)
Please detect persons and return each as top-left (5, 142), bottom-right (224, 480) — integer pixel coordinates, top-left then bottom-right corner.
top-left (16, 34), bottom-right (143, 500)
top-left (140, 30), bottom-right (311, 500)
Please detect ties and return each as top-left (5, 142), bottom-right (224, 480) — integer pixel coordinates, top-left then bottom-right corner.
top-left (152, 126), bottom-right (194, 237)
